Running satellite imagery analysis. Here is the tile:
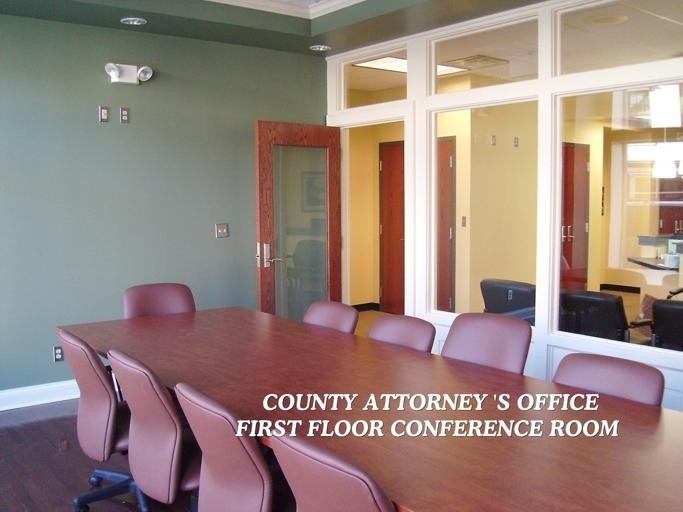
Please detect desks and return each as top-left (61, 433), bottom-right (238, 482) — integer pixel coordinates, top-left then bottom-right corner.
top-left (54, 304), bottom-right (682, 511)
top-left (627, 254), bottom-right (678, 271)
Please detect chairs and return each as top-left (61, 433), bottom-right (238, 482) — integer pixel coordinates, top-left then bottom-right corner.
top-left (478, 278), bottom-right (533, 323)
top-left (298, 301), bottom-right (359, 336)
top-left (365, 312), bottom-right (437, 356)
top-left (262, 420), bottom-right (396, 512)
top-left (118, 282), bottom-right (198, 323)
top-left (103, 347), bottom-right (201, 512)
top-left (53, 324), bottom-right (191, 512)
top-left (560, 285), bottom-right (654, 346)
top-left (282, 239), bottom-right (324, 297)
top-left (649, 300), bottom-right (683, 351)
top-left (438, 310), bottom-right (532, 377)
top-left (168, 377), bottom-right (291, 512)
top-left (551, 352), bottom-right (665, 408)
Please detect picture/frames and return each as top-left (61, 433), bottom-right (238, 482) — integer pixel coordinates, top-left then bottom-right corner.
top-left (301, 170), bottom-right (324, 214)
